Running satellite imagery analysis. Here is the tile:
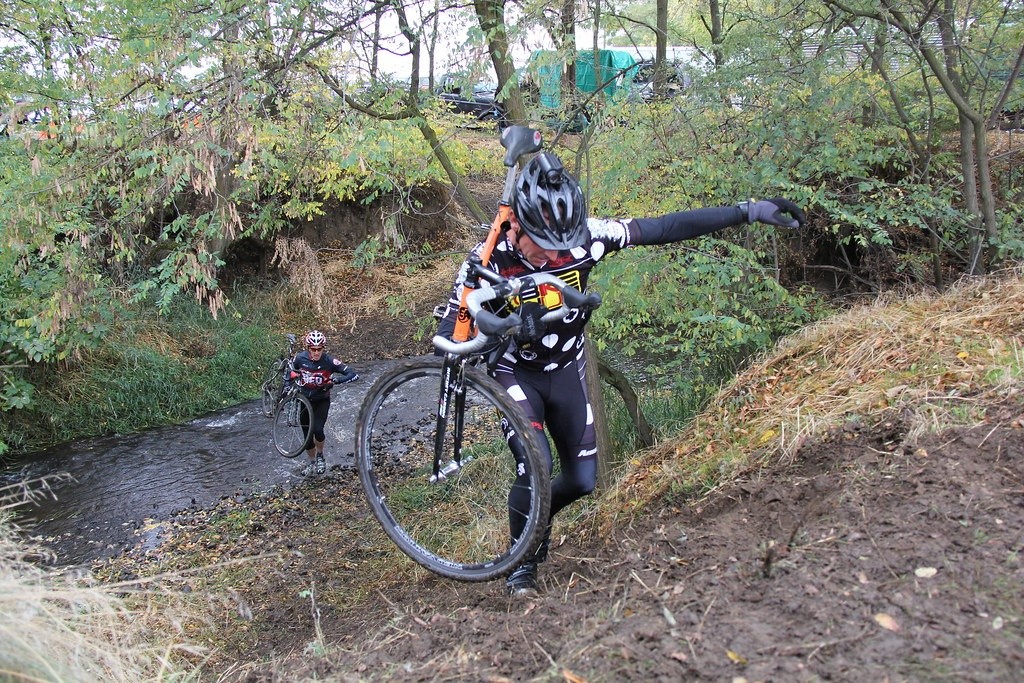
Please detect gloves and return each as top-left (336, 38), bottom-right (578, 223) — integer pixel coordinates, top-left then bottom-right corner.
top-left (749, 197), bottom-right (807, 228)
top-left (514, 304), bottom-right (549, 342)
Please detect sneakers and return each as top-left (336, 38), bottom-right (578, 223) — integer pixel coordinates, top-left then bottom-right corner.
top-left (506, 559), bottom-right (537, 598)
top-left (316, 456), bottom-right (325, 474)
top-left (301, 461), bottom-right (316, 476)
top-left (540, 518), bottom-right (551, 564)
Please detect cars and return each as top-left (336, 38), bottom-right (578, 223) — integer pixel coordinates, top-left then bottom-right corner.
top-left (401, 76), bottom-right (437, 93)
top-left (417, 84), bottom-right (504, 121)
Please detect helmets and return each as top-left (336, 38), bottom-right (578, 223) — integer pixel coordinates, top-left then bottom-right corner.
top-left (306, 330), bottom-right (326, 349)
top-left (513, 153), bottom-right (587, 250)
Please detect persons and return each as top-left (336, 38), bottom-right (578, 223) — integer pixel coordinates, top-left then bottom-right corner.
top-left (289, 331), bottom-right (359, 475)
top-left (438, 175), bottom-right (806, 587)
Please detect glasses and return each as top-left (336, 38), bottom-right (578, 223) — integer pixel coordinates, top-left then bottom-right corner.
top-left (309, 347), bottom-right (324, 351)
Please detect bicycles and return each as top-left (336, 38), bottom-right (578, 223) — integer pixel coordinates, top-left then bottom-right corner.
top-left (262, 334), bottom-right (324, 458)
top-left (354, 125), bottom-right (601, 583)
